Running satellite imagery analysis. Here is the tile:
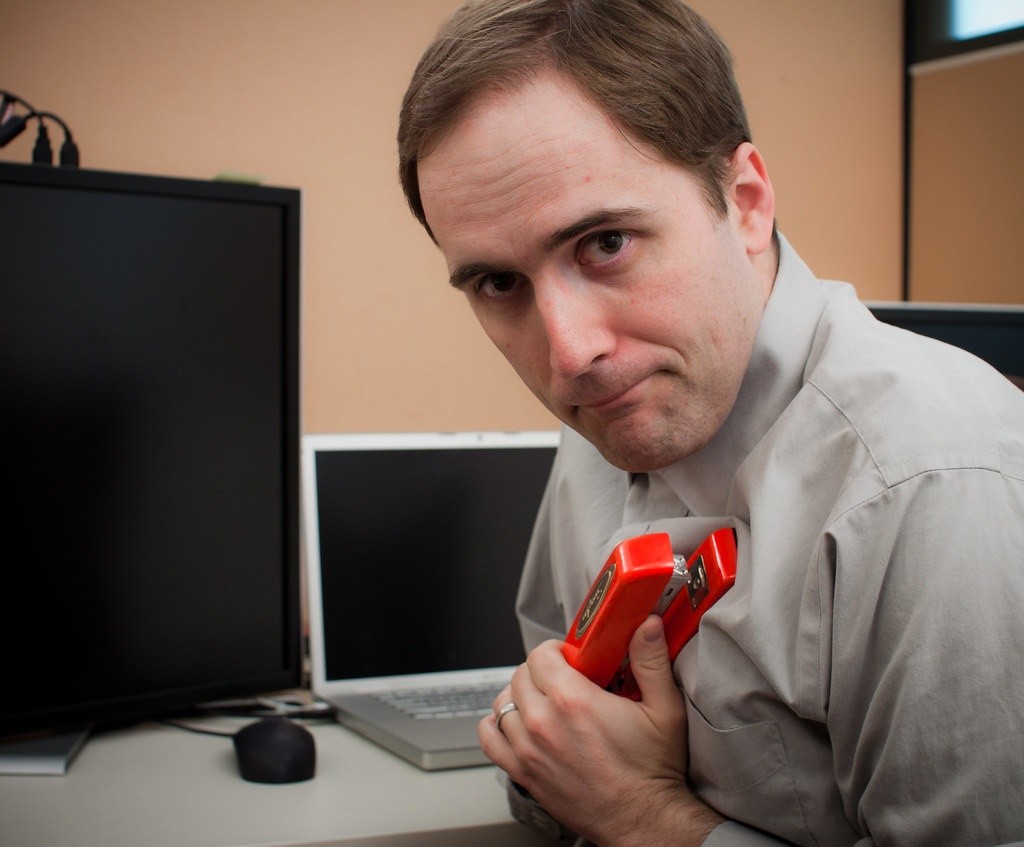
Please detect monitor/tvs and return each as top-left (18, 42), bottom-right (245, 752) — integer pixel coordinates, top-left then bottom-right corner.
top-left (0, 164), bottom-right (308, 773)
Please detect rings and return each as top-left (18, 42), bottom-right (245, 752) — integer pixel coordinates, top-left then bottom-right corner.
top-left (495, 703), bottom-right (518, 730)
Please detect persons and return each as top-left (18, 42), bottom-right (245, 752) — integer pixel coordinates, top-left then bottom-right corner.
top-left (400, 0), bottom-right (1024, 847)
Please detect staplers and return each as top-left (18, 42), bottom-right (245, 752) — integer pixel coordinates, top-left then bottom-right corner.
top-left (559, 527), bottom-right (737, 702)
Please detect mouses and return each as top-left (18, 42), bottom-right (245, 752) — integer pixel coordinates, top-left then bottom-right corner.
top-left (233, 717), bottom-right (313, 784)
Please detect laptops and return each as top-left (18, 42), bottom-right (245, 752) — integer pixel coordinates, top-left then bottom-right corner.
top-left (300, 428), bottom-right (566, 769)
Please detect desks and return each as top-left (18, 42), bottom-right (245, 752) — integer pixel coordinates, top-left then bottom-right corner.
top-left (1, 710), bottom-right (554, 847)
